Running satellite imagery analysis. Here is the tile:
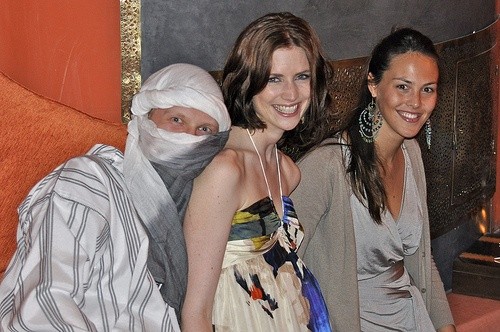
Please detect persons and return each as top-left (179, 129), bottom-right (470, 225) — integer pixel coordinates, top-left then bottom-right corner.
top-left (179, 12), bottom-right (337, 332)
top-left (0, 64), bottom-right (231, 332)
top-left (289, 26), bottom-right (456, 332)
